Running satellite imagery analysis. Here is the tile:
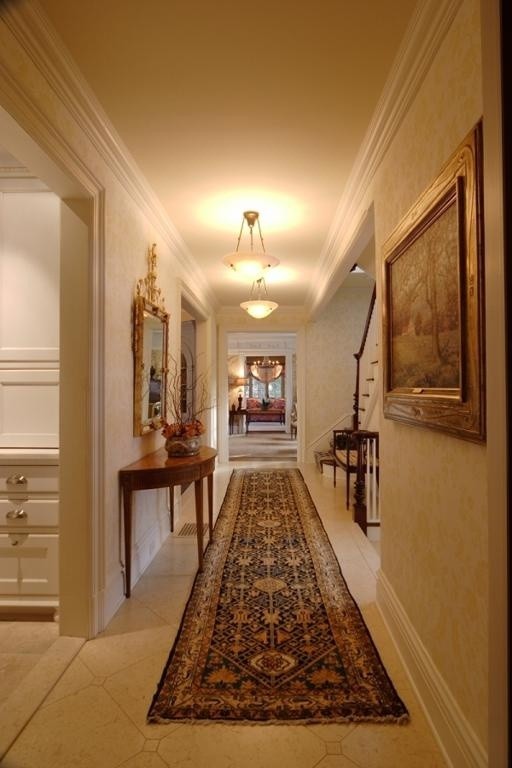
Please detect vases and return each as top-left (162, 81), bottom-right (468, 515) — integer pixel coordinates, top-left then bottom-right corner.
top-left (163, 438), bottom-right (203, 459)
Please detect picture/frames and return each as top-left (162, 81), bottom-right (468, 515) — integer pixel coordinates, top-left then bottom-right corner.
top-left (375, 110), bottom-right (489, 451)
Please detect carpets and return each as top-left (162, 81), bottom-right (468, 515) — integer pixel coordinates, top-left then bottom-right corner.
top-left (143, 464), bottom-right (413, 733)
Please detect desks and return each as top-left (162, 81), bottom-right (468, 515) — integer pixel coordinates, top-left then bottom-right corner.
top-left (230, 409), bottom-right (249, 437)
top-left (116, 441), bottom-right (218, 600)
top-left (246, 409), bottom-right (285, 427)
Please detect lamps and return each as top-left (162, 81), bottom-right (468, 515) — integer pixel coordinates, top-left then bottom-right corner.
top-left (233, 377), bottom-right (250, 408)
top-left (222, 210), bottom-right (281, 321)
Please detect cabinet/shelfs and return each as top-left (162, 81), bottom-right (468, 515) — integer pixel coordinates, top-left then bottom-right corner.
top-left (0, 463), bottom-right (60, 621)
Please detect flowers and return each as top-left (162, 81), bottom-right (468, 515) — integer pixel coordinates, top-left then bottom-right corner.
top-left (143, 415), bottom-right (206, 440)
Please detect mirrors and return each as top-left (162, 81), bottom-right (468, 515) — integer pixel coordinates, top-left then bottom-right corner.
top-left (130, 295), bottom-right (170, 437)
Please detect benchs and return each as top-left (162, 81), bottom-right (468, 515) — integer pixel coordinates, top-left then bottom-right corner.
top-left (332, 430), bottom-right (380, 512)
